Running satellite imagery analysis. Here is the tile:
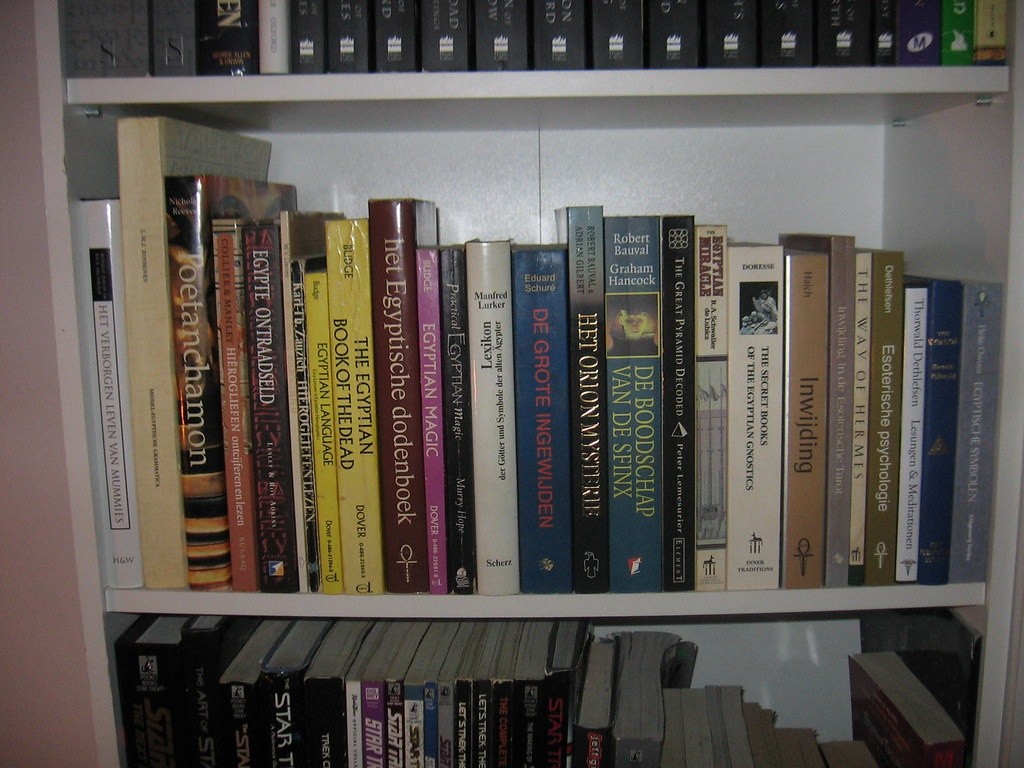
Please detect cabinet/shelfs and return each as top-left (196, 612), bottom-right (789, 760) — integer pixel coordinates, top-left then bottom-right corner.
top-left (34, 0), bottom-right (1024, 768)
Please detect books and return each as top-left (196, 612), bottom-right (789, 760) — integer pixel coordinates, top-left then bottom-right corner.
top-left (71, 114), bottom-right (995, 599)
top-left (65, 0), bottom-right (1012, 77)
top-left (112, 612), bottom-right (970, 768)
